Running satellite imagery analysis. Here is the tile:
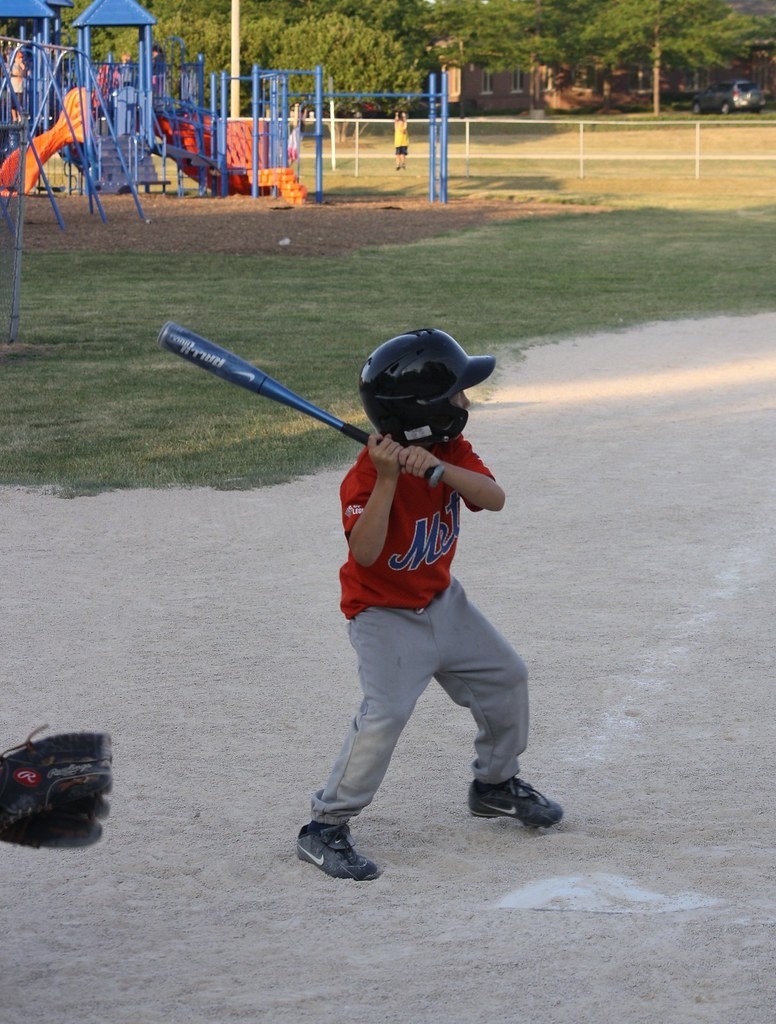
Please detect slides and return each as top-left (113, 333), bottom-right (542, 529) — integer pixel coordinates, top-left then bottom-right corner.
top-left (1, 82), bottom-right (90, 199)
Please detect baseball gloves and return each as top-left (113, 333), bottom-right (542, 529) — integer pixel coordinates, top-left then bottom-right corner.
top-left (0, 722), bottom-right (120, 854)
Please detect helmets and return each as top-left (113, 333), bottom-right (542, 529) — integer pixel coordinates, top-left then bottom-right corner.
top-left (358, 328), bottom-right (496, 448)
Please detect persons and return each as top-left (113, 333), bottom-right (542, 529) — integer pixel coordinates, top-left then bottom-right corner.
top-left (288, 105), bottom-right (308, 166)
top-left (296, 327), bottom-right (565, 883)
top-left (395, 112), bottom-right (408, 172)
top-left (8, 51), bottom-right (30, 121)
top-left (152, 45), bottom-right (167, 92)
top-left (119, 52), bottom-right (132, 85)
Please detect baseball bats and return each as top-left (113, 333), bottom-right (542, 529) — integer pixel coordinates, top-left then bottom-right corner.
top-left (157, 321), bottom-right (444, 483)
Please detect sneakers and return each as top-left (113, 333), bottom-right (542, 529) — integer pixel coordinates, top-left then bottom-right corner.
top-left (468, 775), bottom-right (563, 829)
top-left (297, 821), bottom-right (377, 880)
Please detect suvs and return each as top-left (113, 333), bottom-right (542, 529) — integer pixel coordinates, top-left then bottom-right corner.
top-left (692, 78), bottom-right (766, 116)
